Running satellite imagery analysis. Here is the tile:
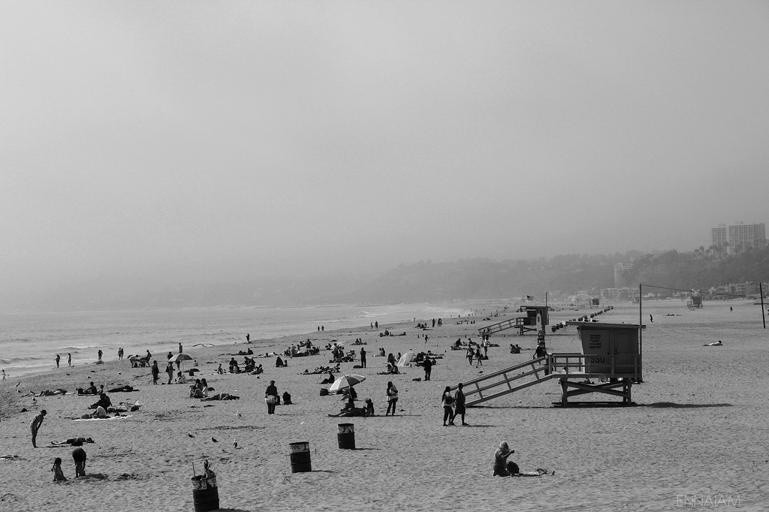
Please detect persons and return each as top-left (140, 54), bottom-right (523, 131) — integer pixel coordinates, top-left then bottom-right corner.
top-left (702, 341), bottom-right (723, 346)
top-left (29, 409), bottom-right (47, 448)
top-left (50, 437), bottom-right (93, 445)
top-left (533, 345), bottom-right (550, 361)
top-left (39, 317), bottom-right (491, 428)
top-left (509, 342), bottom-right (521, 353)
top-left (492, 440), bottom-right (515, 477)
top-left (72, 446), bottom-right (86, 477)
top-left (51, 457), bottom-right (67, 482)
top-left (2, 370), bottom-right (6, 382)
top-left (15, 381), bottom-right (24, 393)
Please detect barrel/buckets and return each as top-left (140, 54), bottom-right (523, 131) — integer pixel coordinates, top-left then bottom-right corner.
top-left (191, 475), bottom-right (219, 512)
top-left (337, 423), bottom-right (355, 449)
top-left (288, 441), bottom-right (311, 473)
